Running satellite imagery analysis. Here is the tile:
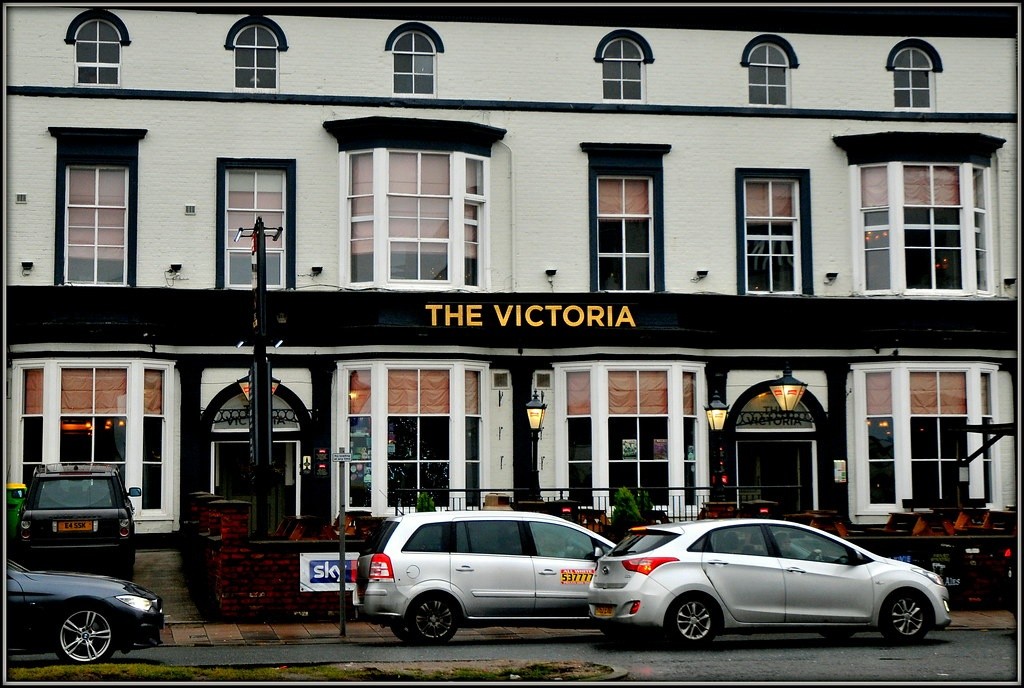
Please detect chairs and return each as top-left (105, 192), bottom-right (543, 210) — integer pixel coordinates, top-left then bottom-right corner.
top-left (725, 531), bottom-right (738, 549)
top-left (88, 480), bottom-right (110, 506)
top-left (750, 529), bottom-right (764, 545)
top-left (45, 480), bottom-right (60, 488)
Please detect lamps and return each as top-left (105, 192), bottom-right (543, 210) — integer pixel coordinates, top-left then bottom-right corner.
top-left (312, 267), bottom-right (322, 273)
top-left (1004, 278), bottom-right (1017, 285)
top-left (826, 272), bottom-right (839, 280)
top-left (22, 261), bottom-right (33, 270)
top-left (770, 360), bottom-right (808, 411)
top-left (697, 271), bottom-right (708, 278)
top-left (546, 270), bottom-right (556, 276)
top-left (171, 264), bottom-right (181, 272)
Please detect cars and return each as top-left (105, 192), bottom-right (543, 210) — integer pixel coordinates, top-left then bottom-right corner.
top-left (7, 559), bottom-right (164, 665)
top-left (586, 519), bottom-right (952, 649)
top-left (351, 509), bottom-right (618, 645)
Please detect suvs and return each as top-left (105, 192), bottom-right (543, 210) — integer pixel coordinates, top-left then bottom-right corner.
top-left (17, 464), bottom-right (141, 580)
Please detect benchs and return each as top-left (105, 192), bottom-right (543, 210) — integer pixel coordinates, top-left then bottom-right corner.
top-left (271, 510), bottom-right (388, 541)
top-left (784, 512), bottom-right (849, 537)
top-left (883, 503), bottom-right (1016, 535)
top-left (577, 500), bottom-right (778, 537)
